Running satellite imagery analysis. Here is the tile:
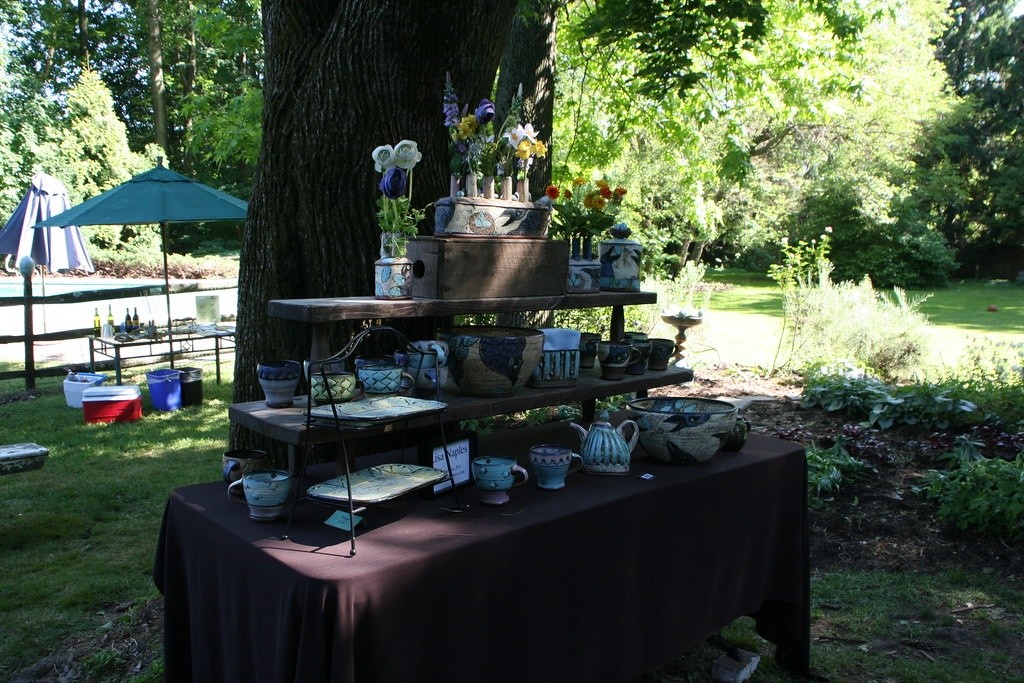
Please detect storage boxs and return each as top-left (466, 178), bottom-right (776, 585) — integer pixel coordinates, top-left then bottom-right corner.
top-left (63, 373), bottom-right (142, 421)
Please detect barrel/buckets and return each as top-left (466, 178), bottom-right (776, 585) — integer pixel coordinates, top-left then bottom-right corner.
top-left (64, 372), bottom-right (107, 408)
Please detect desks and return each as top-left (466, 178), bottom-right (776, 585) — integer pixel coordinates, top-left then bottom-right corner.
top-left (151, 411), bottom-right (809, 683)
top-left (84, 324), bottom-right (235, 385)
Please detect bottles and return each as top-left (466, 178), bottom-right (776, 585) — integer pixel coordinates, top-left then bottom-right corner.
top-left (94, 308), bottom-right (101, 337)
top-left (133, 307), bottom-right (139, 330)
top-left (68, 368), bottom-right (88, 382)
top-left (147, 320), bottom-right (157, 338)
top-left (108, 304), bottom-right (115, 336)
top-left (125, 308), bottom-right (131, 333)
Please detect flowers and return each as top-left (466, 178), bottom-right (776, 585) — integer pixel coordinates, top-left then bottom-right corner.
top-left (545, 171), bottom-right (625, 240)
top-left (440, 71), bottom-right (550, 180)
top-left (372, 139), bottom-right (433, 257)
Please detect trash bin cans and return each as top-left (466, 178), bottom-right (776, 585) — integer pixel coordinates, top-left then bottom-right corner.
top-left (146, 369), bottom-right (182, 411)
top-left (176, 367), bottom-right (203, 406)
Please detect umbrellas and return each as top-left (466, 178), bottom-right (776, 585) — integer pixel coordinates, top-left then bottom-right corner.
top-left (0, 171), bottom-right (94, 331)
top-left (32, 156), bottom-right (248, 369)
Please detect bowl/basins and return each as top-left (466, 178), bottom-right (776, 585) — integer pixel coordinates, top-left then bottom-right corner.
top-left (435, 326), bottom-right (544, 396)
top-left (626, 397), bottom-right (751, 464)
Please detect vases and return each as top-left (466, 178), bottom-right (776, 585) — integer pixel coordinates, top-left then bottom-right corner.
top-left (375, 230), bottom-right (414, 299)
top-left (568, 235), bottom-right (602, 295)
top-left (433, 172), bottom-right (552, 239)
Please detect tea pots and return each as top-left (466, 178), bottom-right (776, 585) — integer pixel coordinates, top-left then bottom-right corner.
top-left (569, 410), bottom-right (640, 475)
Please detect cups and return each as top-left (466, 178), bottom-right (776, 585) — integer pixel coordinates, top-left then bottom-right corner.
top-left (647, 338), bottom-right (678, 371)
top-left (598, 340), bottom-right (642, 379)
top-left (311, 373), bottom-right (356, 406)
top-left (579, 333), bottom-right (601, 369)
top-left (598, 242), bottom-right (642, 292)
top-left (620, 338), bottom-right (652, 375)
top-left (140, 323), bottom-right (144, 327)
top-left (355, 339), bottom-right (448, 394)
top-left (528, 445), bottom-right (583, 489)
top-left (472, 456), bottom-right (528, 504)
top-left (103, 323), bottom-right (112, 338)
top-left (623, 332), bottom-right (647, 340)
top-left (222, 449), bottom-right (292, 520)
top-left (374, 257), bottom-right (414, 299)
top-left (256, 360), bottom-right (301, 408)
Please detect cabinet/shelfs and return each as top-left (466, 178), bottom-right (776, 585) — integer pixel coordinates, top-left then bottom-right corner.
top-left (227, 288), bottom-right (694, 482)
top-left (281, 325), bottom-right (471, 557)
